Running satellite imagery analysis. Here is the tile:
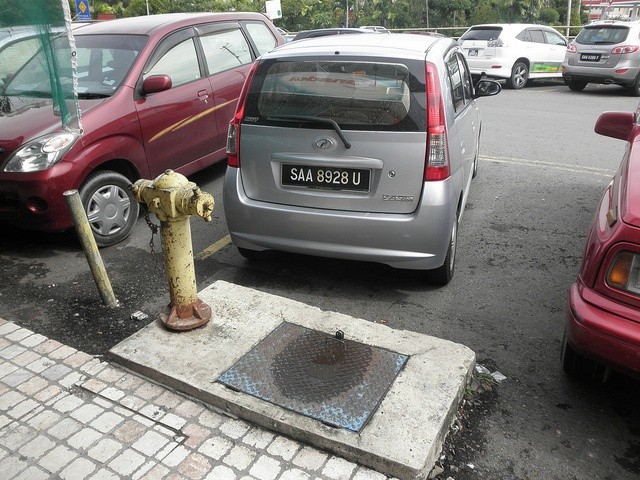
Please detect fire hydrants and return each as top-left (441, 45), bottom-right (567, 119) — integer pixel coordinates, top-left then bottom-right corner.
top-left (128, 170), bottom-right (215, 334)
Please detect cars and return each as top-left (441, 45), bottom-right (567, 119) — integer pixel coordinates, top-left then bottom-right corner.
top-left (457, 23), bottom-right (568, 89)
top-left (278, 27), bottom-right (296, 41)
top-left (0, 11), bottom-right (286, 246)
top-left (0, 23), bottom-right (89, 95)
top-left (361, 26), bottom-right (391, 34)
top-left (561, 106), bottom-right (640, 380)
top-left (292, 28), bottom-right (380, 41)
top-left (562, 20), bottom-right (640, 96)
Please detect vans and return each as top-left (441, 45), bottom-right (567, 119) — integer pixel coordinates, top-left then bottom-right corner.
top-left (224, 28), bottom-right (482, 281)
